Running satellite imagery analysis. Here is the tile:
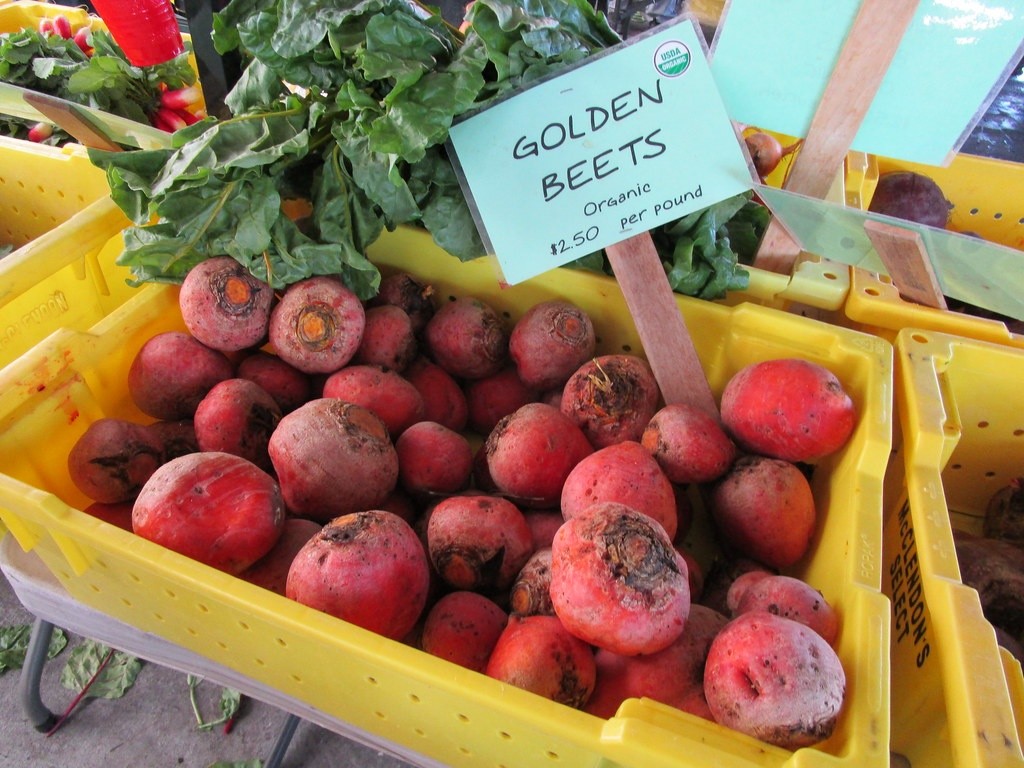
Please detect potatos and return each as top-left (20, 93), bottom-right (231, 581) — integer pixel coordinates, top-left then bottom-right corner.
top-left (717, 357), bottom-right (856, 464)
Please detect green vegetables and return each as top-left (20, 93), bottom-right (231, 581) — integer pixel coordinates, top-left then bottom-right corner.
top-left (82, 0), bottom-right (769, 302)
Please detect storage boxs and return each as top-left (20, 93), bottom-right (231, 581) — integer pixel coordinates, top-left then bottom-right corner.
top-left (0, 0), bottom-right (202, 250)
top-left (0, 224), bottom-right (894, 768)
top-left (819, 147), bottom-right (1024, 348)
top-left (716, 124), bottom-right (850, 319)
top-left (883, 328), bottom-right (1024, 768)
